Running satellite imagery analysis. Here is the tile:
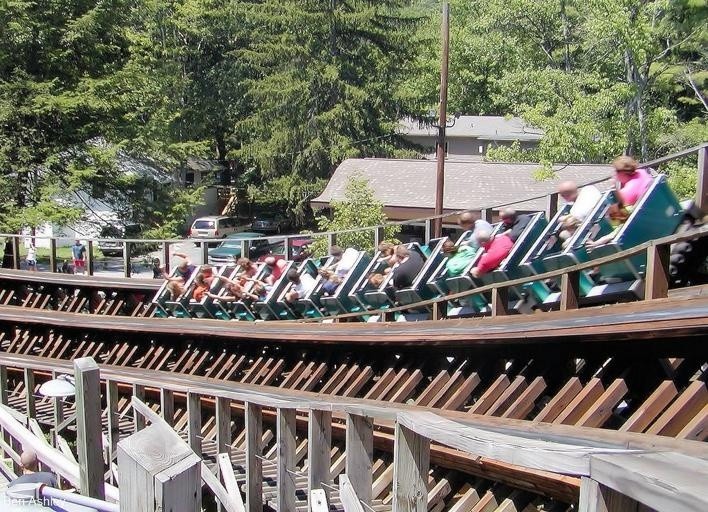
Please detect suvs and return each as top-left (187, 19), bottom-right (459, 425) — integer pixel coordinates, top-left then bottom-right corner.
top-left (189, 209), bottom-right (316, 269)
top-left (97, 222), bottom-right (160, 256)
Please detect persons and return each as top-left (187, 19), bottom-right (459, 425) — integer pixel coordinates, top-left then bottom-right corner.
top-left (150, 258), bottom-right (166, 279)
top-left (556, 181), bottom-right (602, 242)
top-left (71, 240), bottom-right (86, 273)
top-left (25, 244), bottom-right (38, 271)
top-left (57, 259), bottom-right (75, 274)
top-left (584, 205), bottom-right (635, 255)
top-left (605, 154), bottom-right (651, 205)
top-left (156, 207), bottom-right (530, 302)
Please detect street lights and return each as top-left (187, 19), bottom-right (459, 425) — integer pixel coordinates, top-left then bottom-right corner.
top-left (427, 108), bottom-right (463, 239)
top-left (37, 356), bottom-right (106, 502)
